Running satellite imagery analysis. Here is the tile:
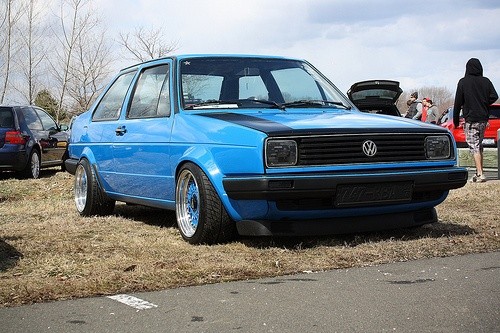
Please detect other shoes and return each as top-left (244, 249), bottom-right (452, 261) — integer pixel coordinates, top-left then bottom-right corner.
top-left (473, 174), bottom-right (486, 183)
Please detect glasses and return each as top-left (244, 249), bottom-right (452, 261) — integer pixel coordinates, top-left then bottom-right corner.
top-left (411, 96), bottom-right (415, 98)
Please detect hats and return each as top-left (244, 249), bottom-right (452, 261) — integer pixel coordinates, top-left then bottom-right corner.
top-left (411, 92), bottom-right (418, 99)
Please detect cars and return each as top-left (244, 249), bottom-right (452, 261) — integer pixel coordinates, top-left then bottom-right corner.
top-left (0, 105), bottom-right (69, 179)
top-left (345, 80), bottom-right (403, 117)
top-left (431, 104), bottom-right (500, 148)
top-left (65, 54), bottom-right (468, 244)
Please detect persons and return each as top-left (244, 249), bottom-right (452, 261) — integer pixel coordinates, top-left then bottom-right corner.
top-left (406, 99), bottom-right (411, 114)
top-left (409, 92), bottom-right (423, 119)
top-left (425, 100), bottom-right (439, 123)
top-left (453, 58), bottom-right (499, 182)
top-left (422, 98), bottom-right (430, 122)
